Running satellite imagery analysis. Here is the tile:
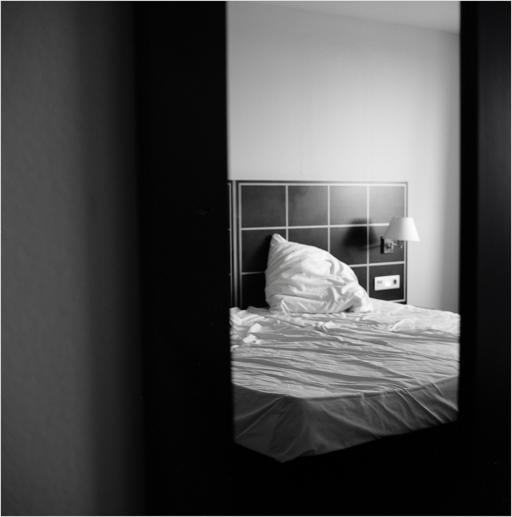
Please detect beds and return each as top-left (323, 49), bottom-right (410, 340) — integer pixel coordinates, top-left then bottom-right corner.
top-left (229, 298), bottom-right (460, 467)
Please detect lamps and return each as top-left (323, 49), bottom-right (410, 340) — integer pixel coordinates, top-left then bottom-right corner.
top-left (381, 217), bottom-right (420, 255)
